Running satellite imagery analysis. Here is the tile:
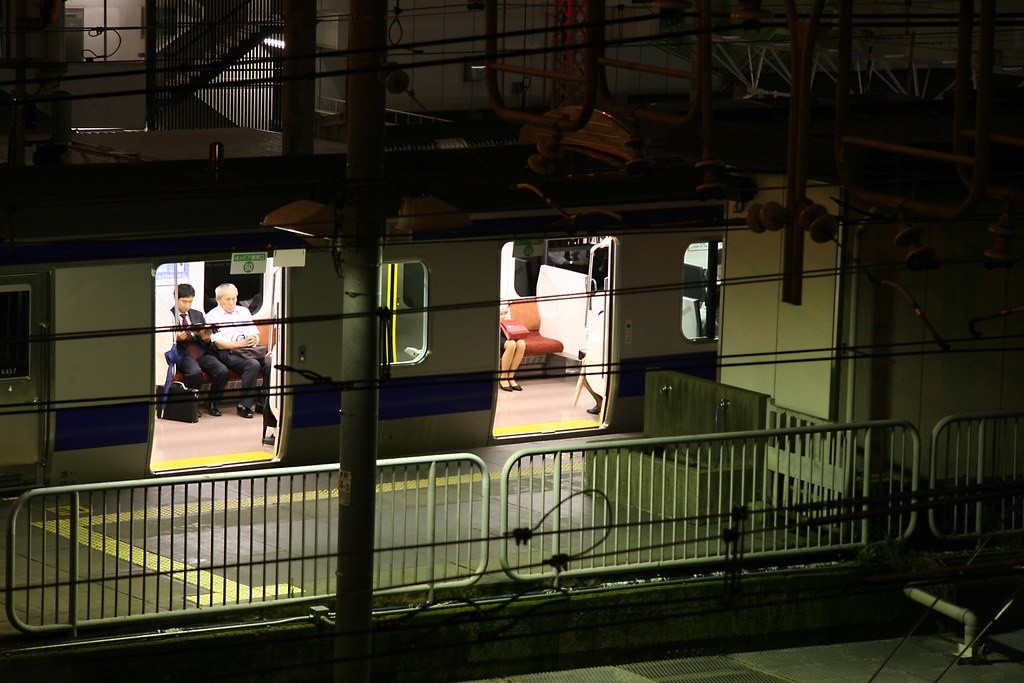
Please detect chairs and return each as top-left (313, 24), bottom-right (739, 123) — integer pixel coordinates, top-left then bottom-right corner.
top-left (681, 296), bottom-right (702, 342)
top-left (536, 264), bottom-right (604, 379)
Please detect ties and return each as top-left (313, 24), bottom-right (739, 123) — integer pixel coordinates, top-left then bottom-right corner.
top-left (179, 314), bottom-right (200, 360)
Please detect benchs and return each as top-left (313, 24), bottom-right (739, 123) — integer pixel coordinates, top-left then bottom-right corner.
top-left (500, 309), bottom-right (563, 377)
top-left (170, 319), bottom-right (270, 406)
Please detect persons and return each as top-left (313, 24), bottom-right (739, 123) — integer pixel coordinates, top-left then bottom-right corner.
top-left (204, 284), bottom-right (271, 419)
top-left (170, 284), bottom-right (228, 417)
top-left (498, 304), bottom-right (526, 392)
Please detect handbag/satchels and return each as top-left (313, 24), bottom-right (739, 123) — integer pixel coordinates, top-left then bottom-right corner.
top-left (500, 320), bottom-right (530, 340)
top-left (231, 331), bottom-right (266, 359)
top-left (156, 381), bottom-right (199, 423)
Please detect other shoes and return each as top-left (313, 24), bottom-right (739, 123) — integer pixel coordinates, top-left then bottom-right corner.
top-left (499, 380), bottom-right (513, 391)
top-left (197, 410), bottom-right (201, 418)
top-left (511, 385), bottom-right (522, 391)
top-left (237, 403), bottom-right (253, 418)
top-left (208, 403), bottom-right (222, 415)
top-left (586, 406), bottom-right (601, 414)
top-left (255, 403), bottom-right (263, 414)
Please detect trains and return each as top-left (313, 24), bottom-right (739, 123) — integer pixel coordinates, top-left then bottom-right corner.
top-left (0, 105), bottom-right (719, 499)
